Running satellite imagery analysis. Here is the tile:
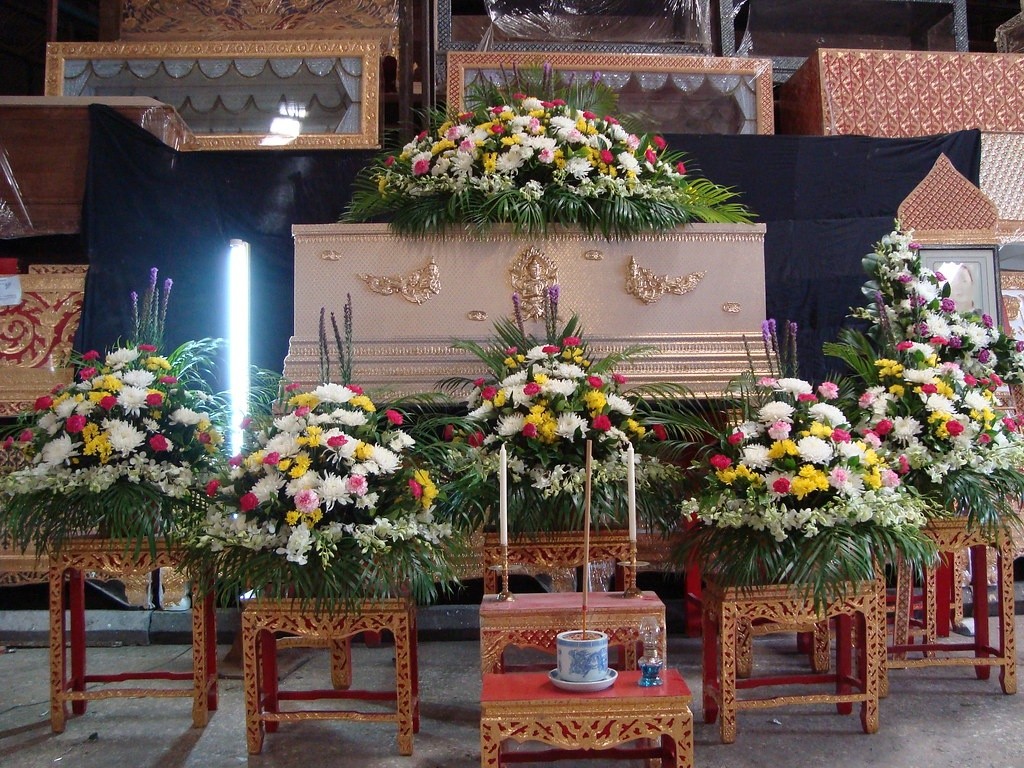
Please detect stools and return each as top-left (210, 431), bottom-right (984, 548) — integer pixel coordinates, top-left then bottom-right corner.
top-left (49, 534), bottom-right (219, 733)
top-left (480, 668), bottom-right (694, 768)
top-left (479, 591), bottom-right (668, 768)
top-left (481, 525), bottom-right (638, 675)
top-left (872, 521), bottom-right (1017, 699)
top-left (242, 597), bottom-right (420, 756)
top-left (701, 573), bottom-right (880, 744)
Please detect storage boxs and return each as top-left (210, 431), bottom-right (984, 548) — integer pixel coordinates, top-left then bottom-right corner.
top-left (779, 47), bottom-right (1024, 139)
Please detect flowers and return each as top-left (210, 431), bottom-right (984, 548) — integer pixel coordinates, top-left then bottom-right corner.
top-left (0, 267), bottom-right (237, 567)
top-left (172, 292), bottom-right (478, 618)
top-left (821, 333), bottom-right (1024, 557)
top-left (337, 63), bottom-right (759, 245)
top-left (845, 218), bottom-right (1024, 385)
top-left (432, 284), bottom-right (705, 549)
top-left (636, 318), bottom-right (955, 624)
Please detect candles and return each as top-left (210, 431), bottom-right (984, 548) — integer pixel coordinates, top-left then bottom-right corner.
top-left (500, 441), bottom-right (509, 544)
top-left (627, 441), bottom-right (636, 540)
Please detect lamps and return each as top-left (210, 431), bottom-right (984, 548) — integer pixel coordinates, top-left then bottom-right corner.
top-left (637, 616), bottom-right (664, 687)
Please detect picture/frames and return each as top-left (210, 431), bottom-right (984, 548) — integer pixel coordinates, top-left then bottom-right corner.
top-left (917, 243), bottom-right (1002, 329)
